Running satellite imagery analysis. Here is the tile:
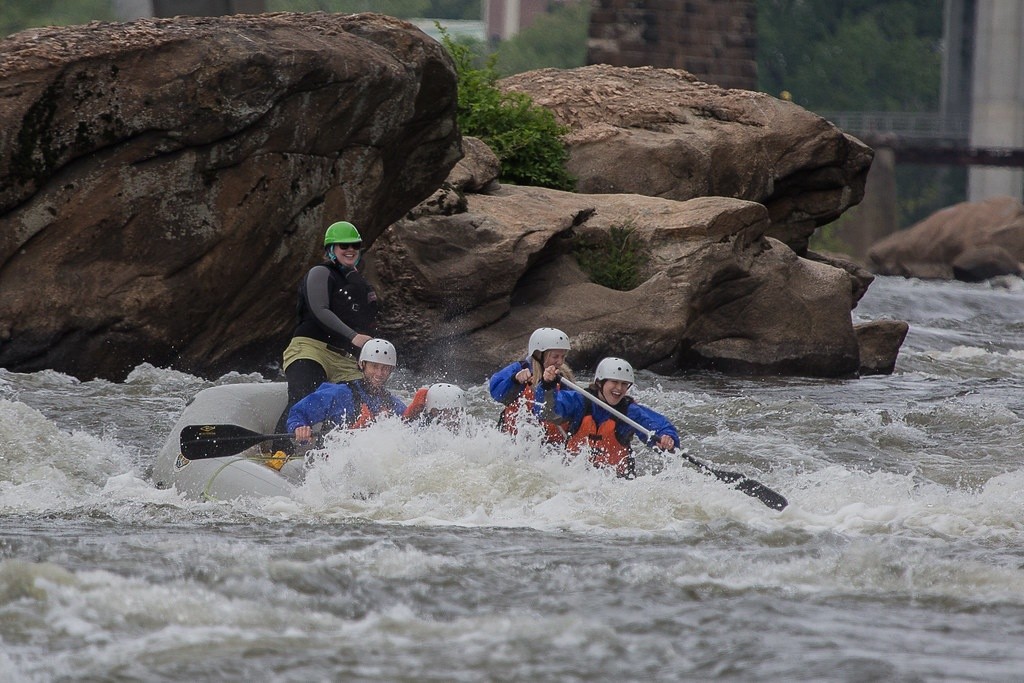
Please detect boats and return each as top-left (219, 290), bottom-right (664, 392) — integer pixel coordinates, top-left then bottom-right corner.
top-left (156, 384), bottom-right (480, 524)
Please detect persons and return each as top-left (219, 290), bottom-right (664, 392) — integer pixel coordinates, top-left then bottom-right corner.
top-left (266, 222), bottom-right (378, 472)
top-left (489, 327), bottom-right (680, 480)
top-left (280, 338), bottom-right (468, 487)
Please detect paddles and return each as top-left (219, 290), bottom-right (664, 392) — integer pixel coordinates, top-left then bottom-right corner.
top-left (178, 420), bottom-right (369, 462)
top-left (552, 368), bottom-right (787, 514)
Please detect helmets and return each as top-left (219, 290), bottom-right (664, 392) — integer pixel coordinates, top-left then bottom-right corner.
top-left (359, 338), bottom-right (396, 372)
top-left (594, 357), bottom-right (635, 385)
top-left (529, 327), bottom-right (571, 358)
top-left (324, 221), bottom-right (362, 250)
top-left (425, 383), bottom-right (470, 415)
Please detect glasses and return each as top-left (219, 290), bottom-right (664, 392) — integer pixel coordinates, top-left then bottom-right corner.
top-left (339, 242), bottom-right (361, 250)
